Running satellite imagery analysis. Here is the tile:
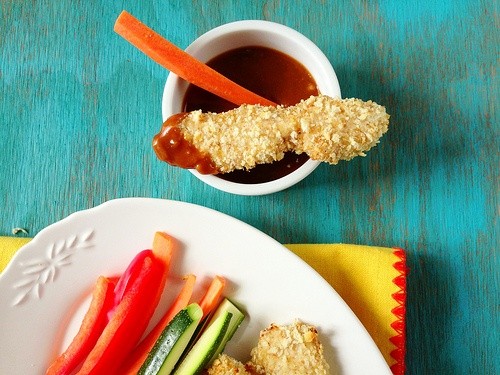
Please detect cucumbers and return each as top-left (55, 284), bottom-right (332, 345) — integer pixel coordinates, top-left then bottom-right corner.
top-left (136, 298), bottom-right (246, 375)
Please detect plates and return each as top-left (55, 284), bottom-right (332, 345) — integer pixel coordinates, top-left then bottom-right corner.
top-left (0, 197), bottom-right (393, 374)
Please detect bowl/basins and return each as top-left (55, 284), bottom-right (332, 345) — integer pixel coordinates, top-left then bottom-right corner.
top-left (161, 20), bottom-right (341, 196)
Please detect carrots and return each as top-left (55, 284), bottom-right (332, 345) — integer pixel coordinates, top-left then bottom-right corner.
top-left (45, 232), bottom-right (227, 375)
top-left (114, 10), bottom-right (278, 107)
